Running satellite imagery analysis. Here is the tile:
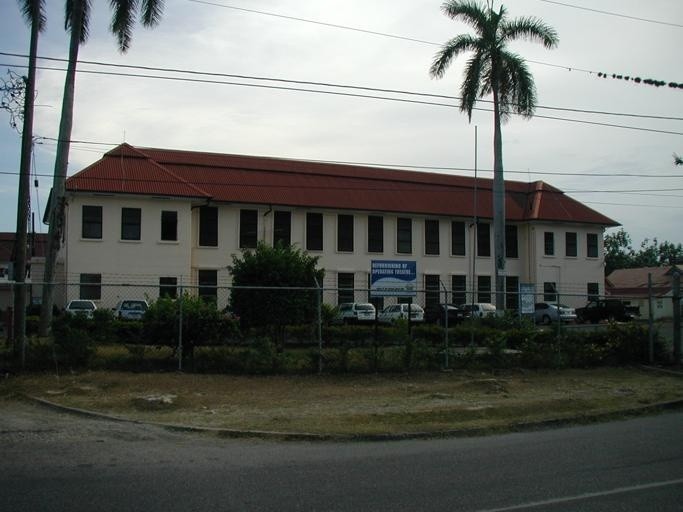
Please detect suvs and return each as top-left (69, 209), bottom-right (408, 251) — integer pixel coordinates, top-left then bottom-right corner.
top-left (573, 298), bottom-right (642, 325)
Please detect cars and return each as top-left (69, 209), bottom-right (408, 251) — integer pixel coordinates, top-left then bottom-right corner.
top-left (377, 303), bottom-right (423, 322)
top-left (420, 302), bottom-right (472, 326)
top-left (526, 301), bottom-right (576, 327)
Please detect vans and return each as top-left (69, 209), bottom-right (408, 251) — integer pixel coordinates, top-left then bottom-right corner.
top-left (456, 301), bottom-right (497, 319)
top-left (337, 303), bottom-right (375, 320)
top-left (112, 298), bottom-right (149, 322)
top-left (63, 298), bottom-right (99, 320)
